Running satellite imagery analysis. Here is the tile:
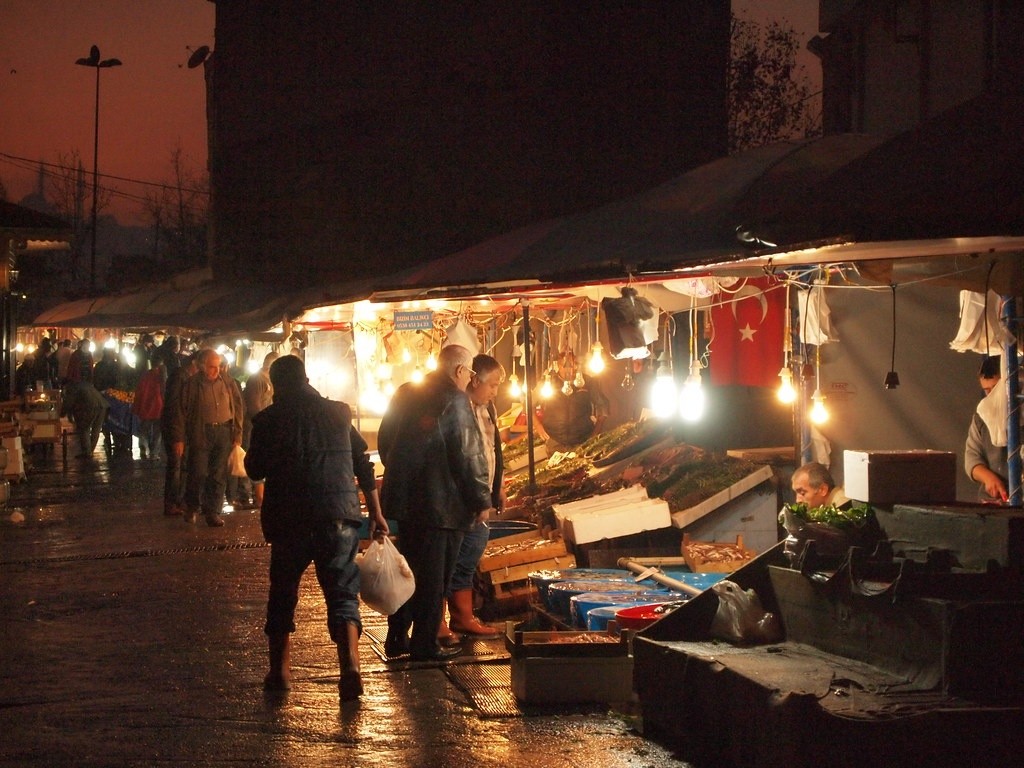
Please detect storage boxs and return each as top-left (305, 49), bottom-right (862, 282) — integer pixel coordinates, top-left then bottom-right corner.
top-left (845, 448), bottom-right (958, 505)
top-left (0, 396), bottom-right (61, 474)
top-left (551, 483), bottom-right (649, 519)
top-left (510, 653), bottom-right (633, 705)
top-left (564, 498), bottom-right (671, 546)
top-left (478, 525), bottom-right (577, 609)
top-left (504, 443), bottom-right (548, 470)
top-left (554, 495), bottom-right (651, 535)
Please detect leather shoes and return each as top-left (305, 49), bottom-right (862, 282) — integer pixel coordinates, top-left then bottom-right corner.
top-left (384, 637), bottom-right (412, 658)
top-left (410, 644), bottom-right (464, 660)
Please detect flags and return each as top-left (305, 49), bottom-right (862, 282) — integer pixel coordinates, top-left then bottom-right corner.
top-left (710, 274), bottom-right (787, 387)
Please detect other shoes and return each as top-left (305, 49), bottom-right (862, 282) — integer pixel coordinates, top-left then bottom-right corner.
top-left (206, 512), bottom-right (225, 527)
top-left (184, 511), bottom-right (197, 524)
top-left (164, 505), bottom-right (184, 515)
top-left (229, 498), bottom-right (263, 511)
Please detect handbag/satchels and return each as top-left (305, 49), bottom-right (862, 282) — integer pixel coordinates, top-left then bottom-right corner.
top-left (356, 535), bottom-right (416, 618)
top-left (707, 581), bottom-right (783, 647)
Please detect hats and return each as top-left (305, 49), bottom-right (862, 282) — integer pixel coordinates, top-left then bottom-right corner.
top-left (140, 334), bottom-right (154, 342)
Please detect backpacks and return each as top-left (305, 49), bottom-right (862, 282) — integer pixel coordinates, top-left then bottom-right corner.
top-left (223, 375), bottom-right (247, 418)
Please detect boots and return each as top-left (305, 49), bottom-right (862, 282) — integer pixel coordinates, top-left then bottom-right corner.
top-left (336, 622), bottom-right (364, 697)
top-left (448, 588), bottom-right (496, 635)
top-left (436, 598), bottom-right (460, 646)
top-left (263, 632), bottom-right (292, 689)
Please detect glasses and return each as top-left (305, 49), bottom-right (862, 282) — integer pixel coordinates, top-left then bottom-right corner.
top-left (466, 367), bottom-right (477, 378)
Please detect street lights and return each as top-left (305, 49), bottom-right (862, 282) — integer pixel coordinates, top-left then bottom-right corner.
top-left (75, 45), bottom-right (121, 296)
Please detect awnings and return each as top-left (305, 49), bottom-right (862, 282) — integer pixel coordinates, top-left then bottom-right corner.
top-left (34, 93), bottom-right (1024, 322)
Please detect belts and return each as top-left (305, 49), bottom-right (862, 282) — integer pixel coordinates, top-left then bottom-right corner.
top-left (206, 421), bottom-right (234, 428)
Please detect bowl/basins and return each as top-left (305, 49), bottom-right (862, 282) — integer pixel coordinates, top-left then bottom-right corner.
top-left (527, 568), bottom-right (730, 630)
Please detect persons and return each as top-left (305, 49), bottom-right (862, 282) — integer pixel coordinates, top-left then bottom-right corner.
top-left (791, 461), bottom-right (849, 508)
top-left (16, 332), bottom-right (281, 525)
top-left (965, 355), bottom-right (1009, 503)
top-left (378, 345), bottom-right (493, 660)
top-left (438, 354), bottom-right (506, 645)
top-left (244, 355), bottom-right (389, 697)
